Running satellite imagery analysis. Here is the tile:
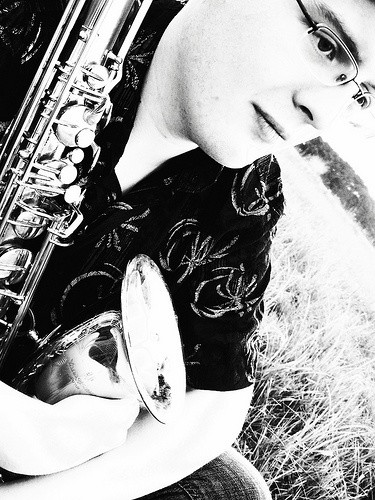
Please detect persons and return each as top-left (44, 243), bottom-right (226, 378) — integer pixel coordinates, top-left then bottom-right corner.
top-left (0, 0), bottom-right (375, 500)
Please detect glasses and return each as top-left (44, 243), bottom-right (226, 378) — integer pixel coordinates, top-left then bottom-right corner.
top-left (297, 0), bottom-right (372, 125)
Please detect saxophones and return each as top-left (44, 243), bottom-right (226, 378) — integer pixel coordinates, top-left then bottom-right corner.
top-left (0, 2), bottom-right (188, 425)
